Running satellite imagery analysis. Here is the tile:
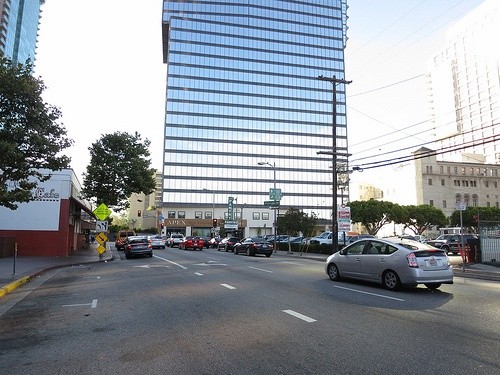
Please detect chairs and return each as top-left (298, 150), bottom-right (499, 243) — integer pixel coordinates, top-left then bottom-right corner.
top-left (370, 246), bottom-right (383, 254)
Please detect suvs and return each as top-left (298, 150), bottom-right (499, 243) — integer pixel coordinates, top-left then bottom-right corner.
top-left (427, 234), bottom-right (478, 256)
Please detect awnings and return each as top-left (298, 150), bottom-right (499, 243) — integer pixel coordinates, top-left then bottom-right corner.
top-left (72, 196), bottom-right (98, 221)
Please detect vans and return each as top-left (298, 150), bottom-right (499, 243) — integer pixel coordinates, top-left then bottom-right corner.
top-left (312, 231), bottom-right (346, 244)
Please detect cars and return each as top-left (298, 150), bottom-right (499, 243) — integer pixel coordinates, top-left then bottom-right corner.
top-left (161, 234), bottom-right (224, 249)
top-left (151, 236), bottom-right (165, 249)
top-left (324, 236), bottom-right (454, 292)
top-left (399, 234), bottom-right (428, 245)
top-left (338, 235), bottom-right (376, 248)
top-left (260, 235), bottom-right (288, 242)
top-left (294, 237), bottom-right (312, 245)
top-left (218, 238), bottom-right (240, 252)
top-left (277, 236), bottom-right (305, 242)
top-left (124, 236), bottom-right (152, 258)
top-left (179, 236), bottom-right (203, 251)
top-left (233, 237), bottom-right (273, 258)
top-left (114, 231), bottom-right (137, 250)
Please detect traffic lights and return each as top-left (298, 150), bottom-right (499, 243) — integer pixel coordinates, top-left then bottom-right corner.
top-left (138, 210), bottom-right (141, 217)
top-left (161, 223), bottom-right (164, 229)
top-left (213, 219), bottom-right (217, 228)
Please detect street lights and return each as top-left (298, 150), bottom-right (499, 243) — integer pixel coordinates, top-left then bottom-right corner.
top-left (258, 161), bottom-right (277, 253)
top-left (203, 189), bottom-right (214, 238)
